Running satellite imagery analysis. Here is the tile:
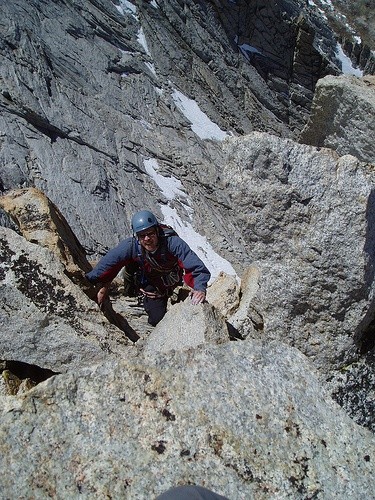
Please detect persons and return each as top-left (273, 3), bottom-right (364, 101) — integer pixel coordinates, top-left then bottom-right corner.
top-left (85, 210), bottom-right (213, 328)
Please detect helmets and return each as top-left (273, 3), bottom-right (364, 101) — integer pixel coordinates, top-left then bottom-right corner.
top-left (131, 210), bottom-right (158, 235)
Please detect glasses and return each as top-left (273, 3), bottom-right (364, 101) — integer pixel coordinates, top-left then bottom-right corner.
top-left (138, 228), bottom-right (156, 238)
